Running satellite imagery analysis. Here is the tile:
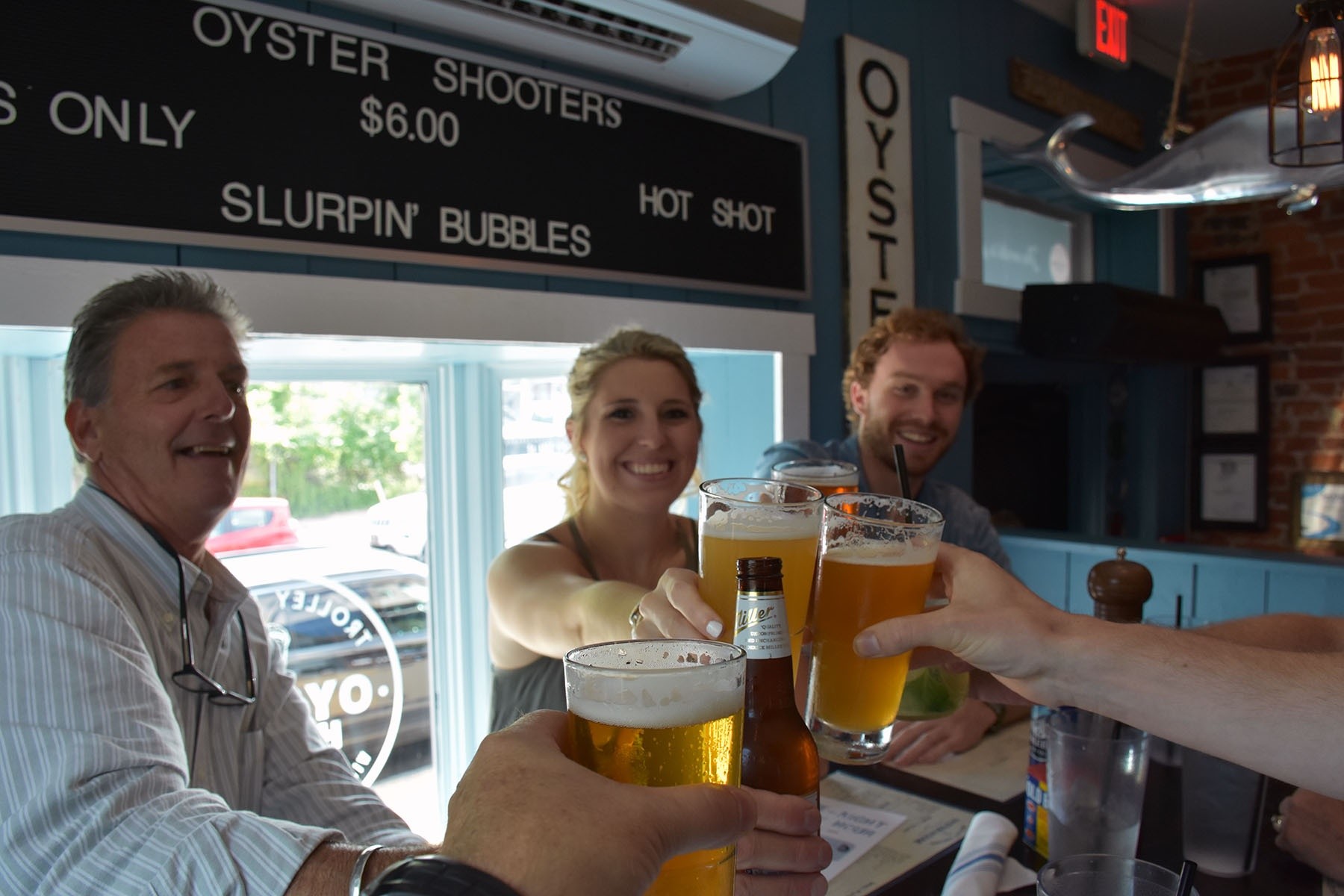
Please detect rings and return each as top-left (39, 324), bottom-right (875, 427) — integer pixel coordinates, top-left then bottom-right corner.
top-left (1270, 815), bottom-right (1284, 831)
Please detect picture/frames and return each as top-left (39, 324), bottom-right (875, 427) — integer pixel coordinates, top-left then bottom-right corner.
top-left (1194, 443), bottom-right (1273, 534)
top-left (1287, 468), bottom-right (1344, 550)
top-left (1194, 356), bottom-right (1267, 441)
top-left (1194, 250), bottom-right (1272, 345)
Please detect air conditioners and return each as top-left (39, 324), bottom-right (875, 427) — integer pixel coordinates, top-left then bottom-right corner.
top-left (205, 0), bottom-right (809, 100)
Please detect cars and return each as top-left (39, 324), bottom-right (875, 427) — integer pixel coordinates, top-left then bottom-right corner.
top-left (364, 376), bottom-right (570, 562)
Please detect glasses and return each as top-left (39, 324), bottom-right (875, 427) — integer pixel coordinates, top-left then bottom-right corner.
top-left (144, 520), bottom-right (260, 709)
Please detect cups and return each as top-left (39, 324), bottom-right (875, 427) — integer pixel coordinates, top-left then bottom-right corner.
top-left (697, 476), bottom-right (824, 722)
top-left (890, 594), bottom-right (970, 721)
top-left (1040, 615), bottom-right (1307, 896)
top-left (561, 633), bottom-right (745, 896)
top-left (772, 459), bottom-right (862, 651)
top-left (807, 489), bottom-right (946, 767)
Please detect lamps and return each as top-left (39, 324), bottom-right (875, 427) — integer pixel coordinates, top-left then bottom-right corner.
top-left (1268, 0), bottom-right (1343, 168)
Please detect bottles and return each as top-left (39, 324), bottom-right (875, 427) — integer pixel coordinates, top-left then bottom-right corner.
top-left (722, 559), bottom-right (822, 894)
top-left (1024, 684), bottom-right (1083, 872)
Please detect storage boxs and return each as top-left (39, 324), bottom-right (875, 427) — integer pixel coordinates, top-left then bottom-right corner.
top-left (1019, 281), bottom-right (1188, 362)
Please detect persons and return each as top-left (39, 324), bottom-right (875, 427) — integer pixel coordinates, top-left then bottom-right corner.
top-left (358, 708), bottom-right (756, 895)
top-left (483, 327), bottom-right (820, 780)
top-left (745, 304), bottom-right (1051, 763)
top-left (1, 258), bottom-right (835, 893)
top-left (1173, 611), bottom-right (1344, 884)
top-left (853, 534), bottom-right (1343, 800)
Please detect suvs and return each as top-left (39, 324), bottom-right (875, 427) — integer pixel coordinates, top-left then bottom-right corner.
top-left (200, 541), bottom-right (435, 761)
top-left (203, 491), bottom-right (299, 559)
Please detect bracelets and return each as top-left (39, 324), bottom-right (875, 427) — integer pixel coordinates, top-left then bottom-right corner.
top-left (359, 853), bottom-right (524, 896)
top-left (350, 842), bottom-right (386, 896)
top-left (628, 603), bottom-right (645, 628)
top-left (981, 700), bottom-right (1007, 731)
